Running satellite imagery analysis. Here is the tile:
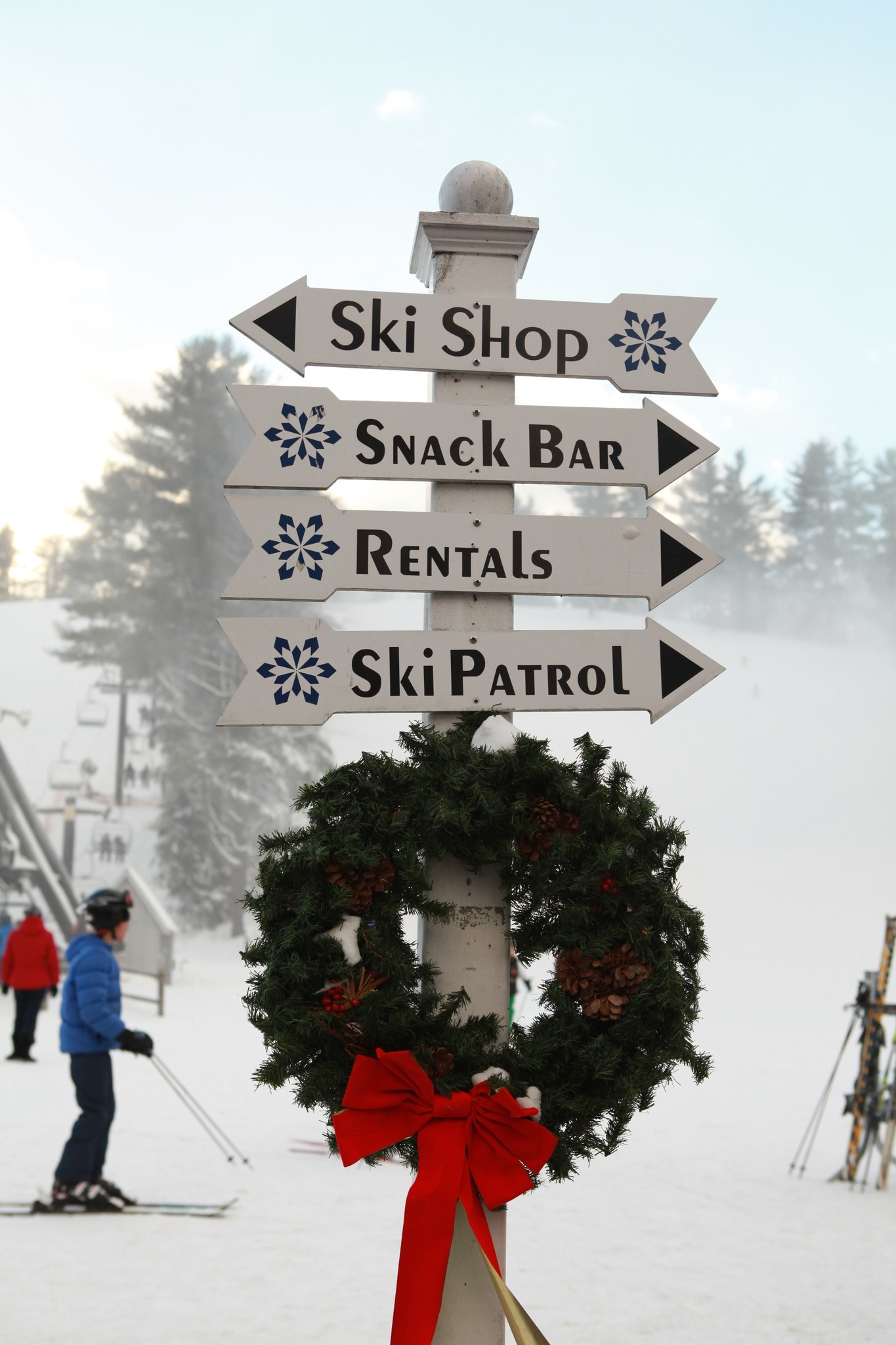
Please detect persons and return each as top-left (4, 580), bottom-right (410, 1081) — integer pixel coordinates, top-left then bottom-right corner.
top-left (0, 907), bottom-right (58, 1062)
top-left (139, 706), bottom-right (148, 723)
top-left (124, 763), bottom-right (150, 789)
top-left (99, 833), bottom-right (125, 863)
top-left (508, 942), bottom-right (530, 1031)
top-left (51, 887), bottom-right (153, 1202)
top-left (0, 915), bottom-right (14, 956)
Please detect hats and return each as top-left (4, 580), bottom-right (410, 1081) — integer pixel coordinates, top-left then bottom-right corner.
top-left (26, 908), bottom-right (40, 916)
top-left (86, 889), bottom-right (129, 929)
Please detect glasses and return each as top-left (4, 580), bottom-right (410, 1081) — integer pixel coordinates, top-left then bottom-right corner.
top-left (120, 891), bottom-right (132, 907)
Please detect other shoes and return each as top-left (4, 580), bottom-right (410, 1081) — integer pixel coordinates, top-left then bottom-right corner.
top-left (20, 1054), bottom-right (35, 1061)
top-left (93, 1177), bottom-right (120, 1197)
top-left (53, 1179), bottom-right (110, 1209)
top-left (7, 1052), bottom-right (21, 1059)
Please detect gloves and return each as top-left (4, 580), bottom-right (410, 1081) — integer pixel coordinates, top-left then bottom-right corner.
top-left (116, 1029), bottom-right (153, 1057)
top-left (3, 985), bottom-right (8, 993)
top-left (51, 986), bottom-right (57, 996)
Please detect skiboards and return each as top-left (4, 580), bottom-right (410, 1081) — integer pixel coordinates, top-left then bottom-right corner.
top-left (839, 915), bottom-right (896, 1182)
top-left (0, 1196), bottom-right (237, 1218)
top-left (875, 1065), bottom-right (896, 1192)
top-left (289, 1136), bottom-right (410, 1165)
